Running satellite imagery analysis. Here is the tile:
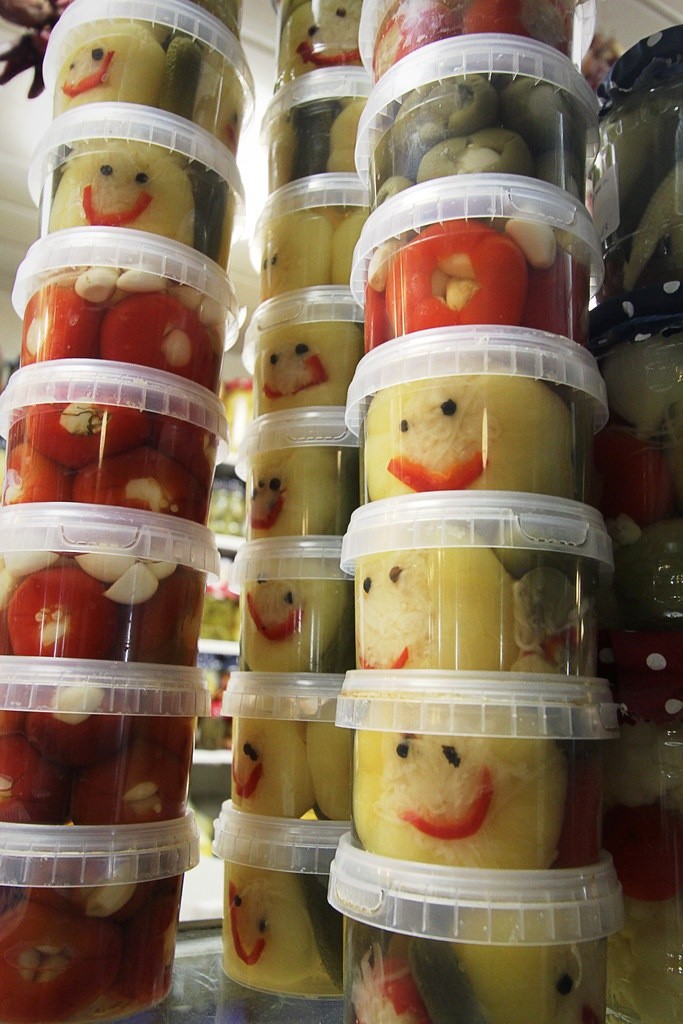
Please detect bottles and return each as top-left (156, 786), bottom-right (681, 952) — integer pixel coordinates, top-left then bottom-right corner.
top-left (589, 25), bottom-right (683, 1024)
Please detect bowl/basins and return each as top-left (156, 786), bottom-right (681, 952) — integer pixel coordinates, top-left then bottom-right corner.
top-left (1, 0), bottom-right (628, 1024)
